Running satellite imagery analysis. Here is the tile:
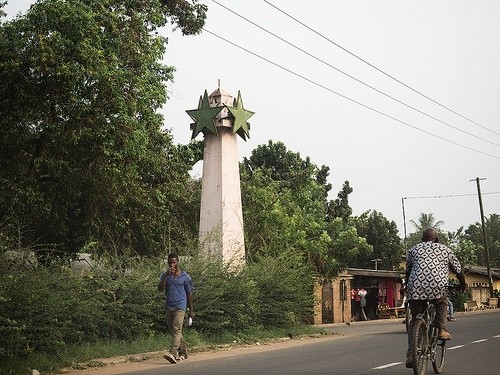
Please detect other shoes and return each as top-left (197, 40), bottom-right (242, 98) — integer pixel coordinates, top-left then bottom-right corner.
top-left (406, 358), bottom-right (414, 368)
top-left (438, 329), bottom-right (452, 340)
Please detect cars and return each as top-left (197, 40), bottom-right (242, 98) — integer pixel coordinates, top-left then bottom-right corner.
top-left (446, 297), bottom-right (456, 321)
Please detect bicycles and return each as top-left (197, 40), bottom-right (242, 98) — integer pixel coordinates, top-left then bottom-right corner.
top-left (402, 285), bottom-right (452, 375)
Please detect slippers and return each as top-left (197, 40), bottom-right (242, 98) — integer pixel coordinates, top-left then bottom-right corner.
top-left (164, 354), bottom-right (177, 364)
top-left (176, 355), bottom-right (189, 362)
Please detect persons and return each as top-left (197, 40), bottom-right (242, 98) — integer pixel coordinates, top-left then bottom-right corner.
top-left (400, 228), bottom-right (468, 368)
top-left (158, 253), bottom-right (195, 364)
